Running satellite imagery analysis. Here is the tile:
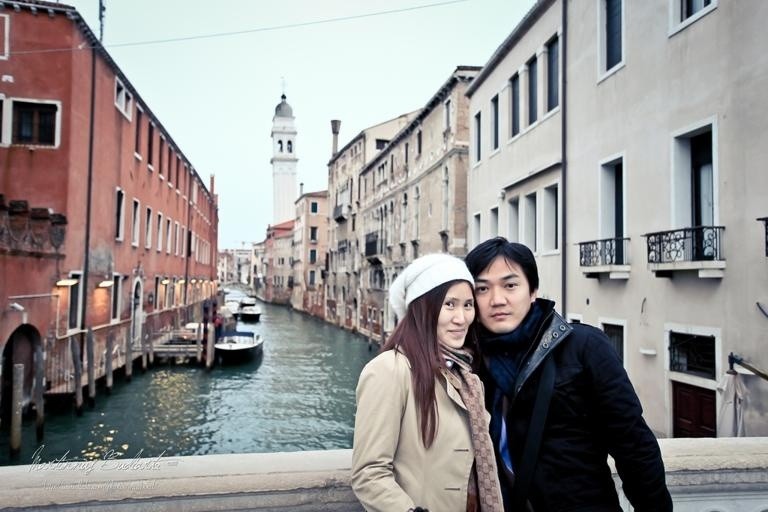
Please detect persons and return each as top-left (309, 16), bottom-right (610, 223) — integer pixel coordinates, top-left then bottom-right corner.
top-left (350, 252), bottom-right (508, 512)
top-left (465, 235), bottom-right (672, 512)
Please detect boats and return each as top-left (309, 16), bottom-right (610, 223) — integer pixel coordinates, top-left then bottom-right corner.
top-left (240, 296), bottom-right (260, 321)
top-left (213, 331), bottom-right (263, 358)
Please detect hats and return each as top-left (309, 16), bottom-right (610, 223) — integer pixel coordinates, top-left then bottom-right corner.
top-left (389, 253), bottom-right (475, 325)
